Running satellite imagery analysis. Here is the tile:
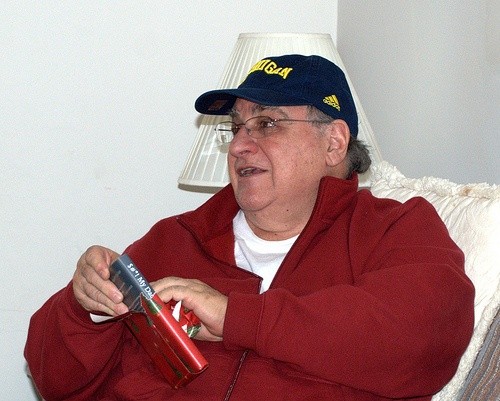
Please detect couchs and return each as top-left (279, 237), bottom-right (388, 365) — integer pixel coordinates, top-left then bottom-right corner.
top-left (352, 173), bottom-right (500, 400)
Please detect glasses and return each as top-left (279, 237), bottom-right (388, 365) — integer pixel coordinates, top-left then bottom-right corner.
top-left (214, 116), bottom-right (331, 143)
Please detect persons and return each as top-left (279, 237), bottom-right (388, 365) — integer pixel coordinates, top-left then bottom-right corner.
top-left (24, 55), bottom-right (474, 401)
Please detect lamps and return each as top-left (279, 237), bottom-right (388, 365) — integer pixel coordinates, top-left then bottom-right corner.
top-left (177, 33), bottom-right (381, 188)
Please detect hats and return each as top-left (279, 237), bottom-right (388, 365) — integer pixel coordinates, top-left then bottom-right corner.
top-left (195, 55), bottom-right (358, 140)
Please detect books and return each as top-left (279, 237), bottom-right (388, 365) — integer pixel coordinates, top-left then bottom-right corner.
top-left (107, 252), bottom-right (210, 387)
